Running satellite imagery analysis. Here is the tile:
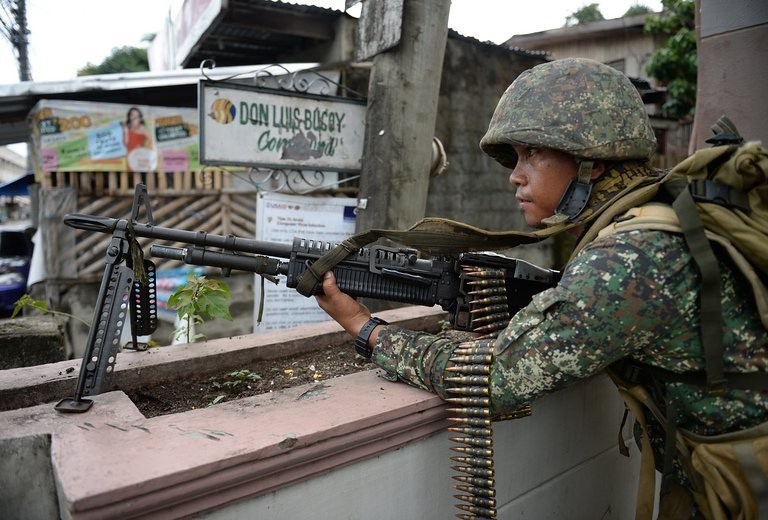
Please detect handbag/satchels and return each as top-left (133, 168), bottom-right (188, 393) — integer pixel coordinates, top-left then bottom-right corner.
top-left (671, 426), bottom-right (768, 520)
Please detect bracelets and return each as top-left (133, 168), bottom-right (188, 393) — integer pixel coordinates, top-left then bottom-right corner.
top-left (355, 317), bottom-right (388, 358)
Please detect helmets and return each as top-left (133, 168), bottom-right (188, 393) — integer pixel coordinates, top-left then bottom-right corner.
top-left (479, 57), bottom-right (656, 162)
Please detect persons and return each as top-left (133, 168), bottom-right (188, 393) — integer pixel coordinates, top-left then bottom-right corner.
top-left (119, 107), bottom-right (153, 171)
top-left (314, 57), bottom-right (768, 520)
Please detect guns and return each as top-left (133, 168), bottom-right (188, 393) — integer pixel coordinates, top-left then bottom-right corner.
top-left (54, 184), bottom-right (563, 413)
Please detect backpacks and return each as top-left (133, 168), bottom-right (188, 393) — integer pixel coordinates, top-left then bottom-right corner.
top-left (559, 116), bottom-right (768, 326)
